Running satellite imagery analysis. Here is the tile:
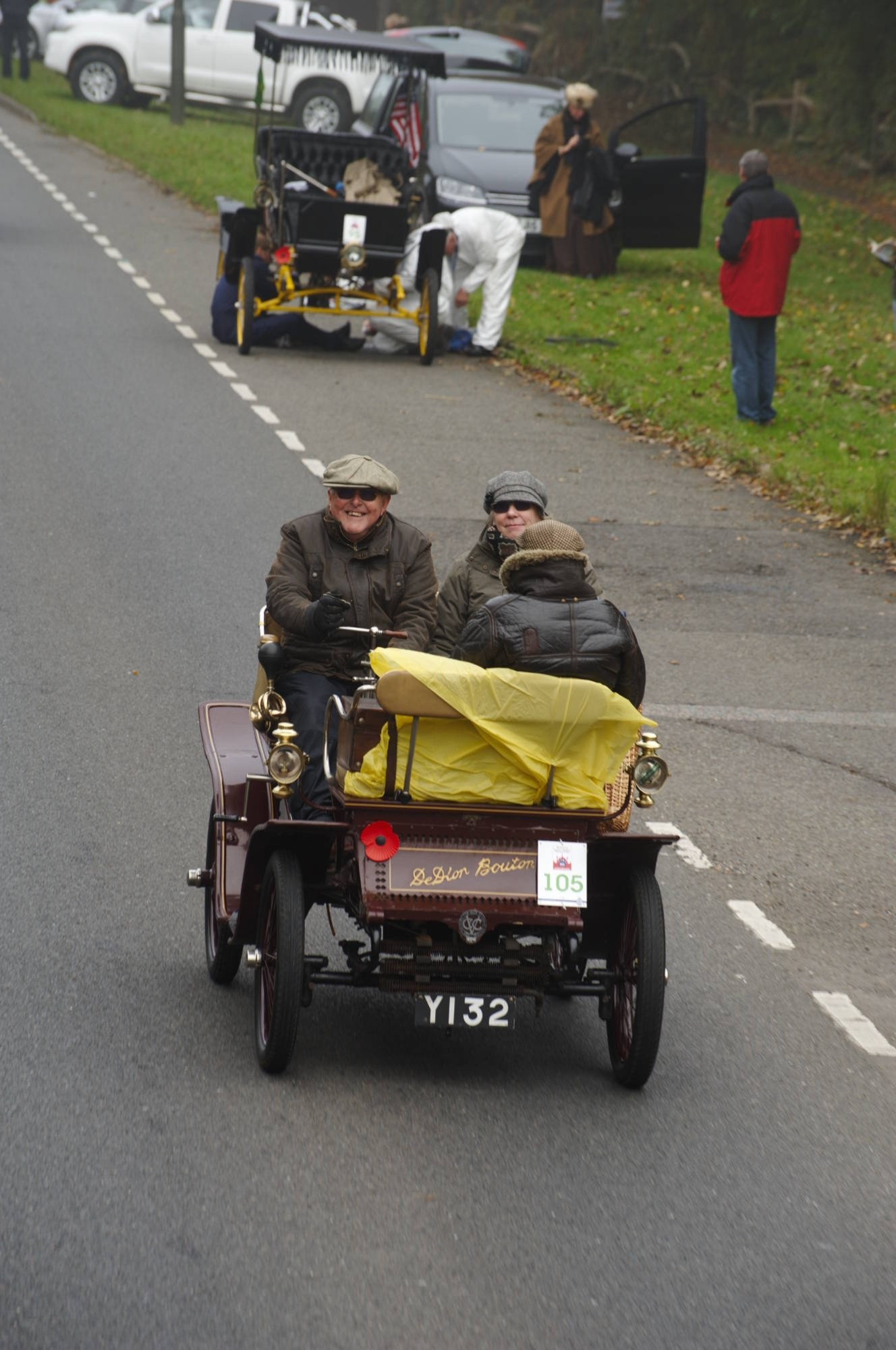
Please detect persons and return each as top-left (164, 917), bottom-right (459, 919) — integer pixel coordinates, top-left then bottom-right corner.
top-left (211, 240), bottom-right (365, 352)
top-left (713, 144), bottom-right (800, 426)
top-left (266, 455), bottom-right (438, 823)
top-left (441, 204), bottom-right (529, 356)
top-left (383, 14), bottom-right (407, 32)
top-left (367, 217), bottom-right (456, 357)
top-left (533, 83), bottom-right (609, 281)
top-left (451, 518), bottom-right (646, 713)
top-left (0, 0), bottom-right (38, 81)
top-left (427, 471), bottom-right (603, 661)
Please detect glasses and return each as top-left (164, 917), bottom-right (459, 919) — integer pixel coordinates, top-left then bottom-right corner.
top-left (337, 489), bottom-right (377, 501)
top-left (493, 502), bottom-right (532, 513)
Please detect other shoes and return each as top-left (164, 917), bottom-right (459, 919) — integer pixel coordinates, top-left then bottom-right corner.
top-left (464, 344), bottom-right (492, 357)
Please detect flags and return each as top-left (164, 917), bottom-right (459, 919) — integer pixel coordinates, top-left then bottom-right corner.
top-left (392, 77), bottom-right (422, 167)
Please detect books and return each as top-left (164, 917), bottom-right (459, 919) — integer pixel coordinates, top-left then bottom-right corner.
top-left (868, 238), bottom-right (895, 265)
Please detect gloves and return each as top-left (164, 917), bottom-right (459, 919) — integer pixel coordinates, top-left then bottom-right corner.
top-left (314, 590), bottom-right (351, 629)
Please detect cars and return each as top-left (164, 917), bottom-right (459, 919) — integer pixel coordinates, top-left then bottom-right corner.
top-left (185, 605), bottom-right (683, 1092)
top-left (347, 24), bottom-right (711, 278)
top-left (216, 24), bottom-right (448, 366)
top-left (10, 0), bottom-right (392, 135)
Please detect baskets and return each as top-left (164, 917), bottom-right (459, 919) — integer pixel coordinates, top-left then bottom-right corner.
top-left (596, 704), bottom-right (642, 832)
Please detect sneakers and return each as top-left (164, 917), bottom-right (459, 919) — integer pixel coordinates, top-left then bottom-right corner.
top-left (329, 337), bottom-right (366, 352)
top-left (330, 320), bottom-right (351, 338)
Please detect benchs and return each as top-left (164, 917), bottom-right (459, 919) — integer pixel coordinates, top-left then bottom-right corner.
top-left (279, 187), bottom-right (409, 255)
top-left (252, 125), bottom-right (410, 202)
top-left (326, 674), bottom-right (628, 820)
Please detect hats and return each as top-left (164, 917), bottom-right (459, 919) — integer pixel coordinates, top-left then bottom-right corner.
top-left (323, 453), bottom-right (399, 495)
top-left (483, 470), bottom-right (548, 514)
top-left (564, 82), bottom-right (599, 110)
top-left (515, 518), bottom-right (585, 552)
